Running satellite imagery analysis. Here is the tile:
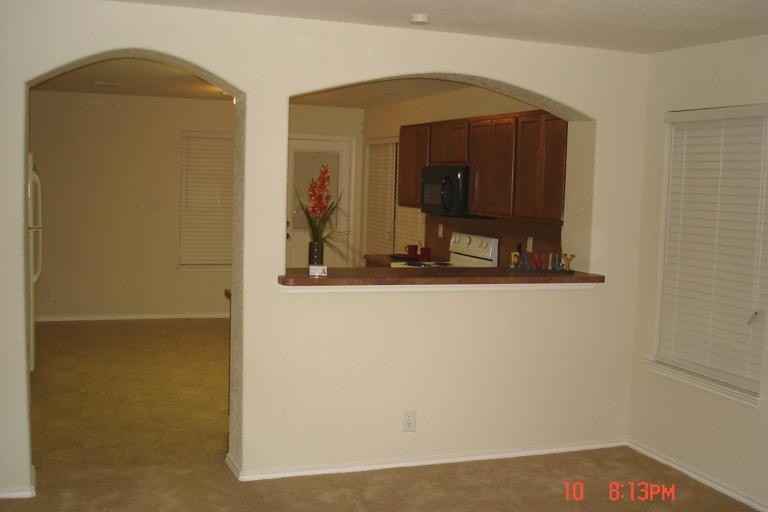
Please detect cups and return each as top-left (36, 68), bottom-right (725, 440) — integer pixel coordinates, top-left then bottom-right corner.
top-left (404, 245), bottom-right (418, 257)
top-left (420, 247), bottom-right (430, 259)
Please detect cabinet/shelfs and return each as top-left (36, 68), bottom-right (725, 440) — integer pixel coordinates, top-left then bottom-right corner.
top-left (396, 109), bottom-right (568, 225)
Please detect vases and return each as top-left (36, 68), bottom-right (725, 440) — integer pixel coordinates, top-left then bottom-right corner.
top-left (306, 239), bottom-right (328, 266)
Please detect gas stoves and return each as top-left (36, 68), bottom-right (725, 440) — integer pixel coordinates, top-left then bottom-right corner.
top-left (390, 252), bottom-right (499, 269)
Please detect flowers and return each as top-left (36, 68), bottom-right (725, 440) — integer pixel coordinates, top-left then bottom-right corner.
top-left (294, 166), bottom-right (357, 264)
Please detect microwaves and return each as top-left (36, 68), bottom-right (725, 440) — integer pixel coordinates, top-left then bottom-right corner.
top-left (419, 165), bottom-right (496, 220)
